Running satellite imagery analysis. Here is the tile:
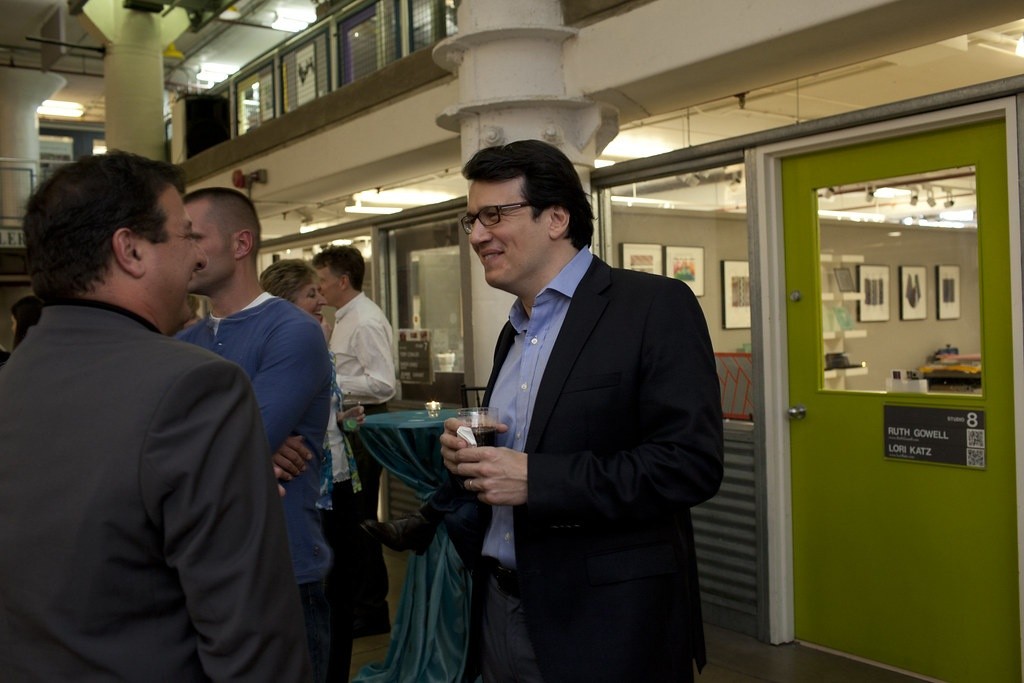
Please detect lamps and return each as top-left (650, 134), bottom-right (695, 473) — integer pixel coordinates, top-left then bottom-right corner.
top-left (679, 172), bottom-right (876, 205)
top-left (908, 187), bottom-right (955, 209)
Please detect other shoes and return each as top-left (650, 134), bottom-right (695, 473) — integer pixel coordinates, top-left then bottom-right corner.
top-left (351, 599), bottom-right (390, 639)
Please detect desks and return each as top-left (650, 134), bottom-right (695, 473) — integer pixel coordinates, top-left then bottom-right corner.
top-left (352, 407), bottom-right (486, 682)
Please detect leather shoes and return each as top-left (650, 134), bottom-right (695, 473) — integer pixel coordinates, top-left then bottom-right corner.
top-left (359, 513), bottom-right (434, 556)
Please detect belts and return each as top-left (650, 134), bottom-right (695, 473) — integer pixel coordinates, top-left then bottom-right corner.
top-left (480, 561), bottom-right (520, 598)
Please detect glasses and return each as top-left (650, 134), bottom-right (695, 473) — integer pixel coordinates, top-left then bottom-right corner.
top-left (460, 200), bottom-right (542, 233)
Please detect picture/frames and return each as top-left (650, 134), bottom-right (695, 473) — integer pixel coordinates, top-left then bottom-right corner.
top-left (934, 264), bottom-right (961, 321)
top-left (834, 268), bottom-right (855, 292)
top-left (620, 242), bottom-right (664, 280)
top-left (899, 267), bottom-right (928, 324)
top-left (722, 262), bottom-right (752, 330)
top-left (664, 245), bottom-right (706, 300)
top-left (855, 264), bottom-right (891, 325)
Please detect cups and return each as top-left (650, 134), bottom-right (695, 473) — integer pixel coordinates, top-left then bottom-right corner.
top-left (340, 402), bottom-right (360, 431)
top-left (457, 407), bottom-right (499, 448)
top-left (437, 354), bottom-right (454, 372)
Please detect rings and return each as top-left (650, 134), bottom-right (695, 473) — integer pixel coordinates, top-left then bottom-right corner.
top-left (469, 476), bottom-right (474, 491)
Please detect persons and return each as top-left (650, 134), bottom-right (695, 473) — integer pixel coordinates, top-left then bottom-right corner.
top-left (0, 148), bottom-right (306, 683)
top-left (10, 295), bottom-right (42, 355)
top-left (263, 259), bottom-right (353, 683)
top-left (0, 347), bottom-right (10, 369)
top-left (440, 139), bottom-right (723, 683)
top-left (169, 187), bottom-right (334, 683)
top-left (184, 292), bottom-right (202, 329)
top-left (360, 475), bottom-right (458, 557)
top-left (314, 242), bottom-right (398, 635)
